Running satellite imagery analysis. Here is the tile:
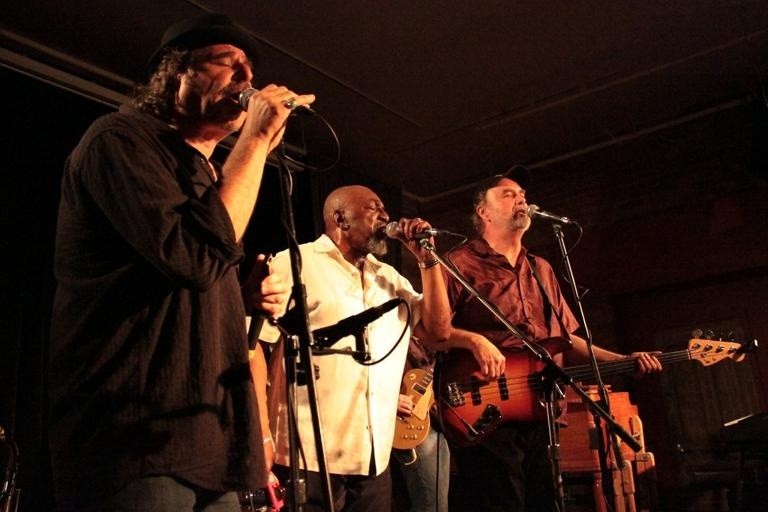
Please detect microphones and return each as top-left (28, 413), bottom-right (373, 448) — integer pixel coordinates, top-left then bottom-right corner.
top-left (527, 204), bottom-right (573, 225)
top-left (239, 88), bottom-right (312, 116)
top-left (385, 221), bottom-right (444, 240)
top-left (311, 298), bottom-right (405, 350)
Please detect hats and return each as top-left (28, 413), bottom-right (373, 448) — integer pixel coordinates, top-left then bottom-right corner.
top-left (148, 13), bottom-right (251, 62)
top-left (472, 164), bottom-right (534, 196)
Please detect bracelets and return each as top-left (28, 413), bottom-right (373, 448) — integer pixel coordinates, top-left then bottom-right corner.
top-left (417, 258), bottom-right (440, 268)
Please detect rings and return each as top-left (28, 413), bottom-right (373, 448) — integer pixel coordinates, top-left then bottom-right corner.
top-left (286, 97), bottom-right (297, 110)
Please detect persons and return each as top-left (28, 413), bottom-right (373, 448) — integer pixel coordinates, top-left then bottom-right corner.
top-left (49, 12), bottom-right (315, 512)
top-left (243, 185), bottom-right (453, 512)
top-left (423, 174), bottom-right (665, 511)
top-left (398, 336), bottom-right (456, 512)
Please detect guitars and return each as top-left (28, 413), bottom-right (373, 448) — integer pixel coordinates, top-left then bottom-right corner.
top-left (392, 354), bottom-right (436, 452)
top-left (433, 326), bottom-right (759, 447)
